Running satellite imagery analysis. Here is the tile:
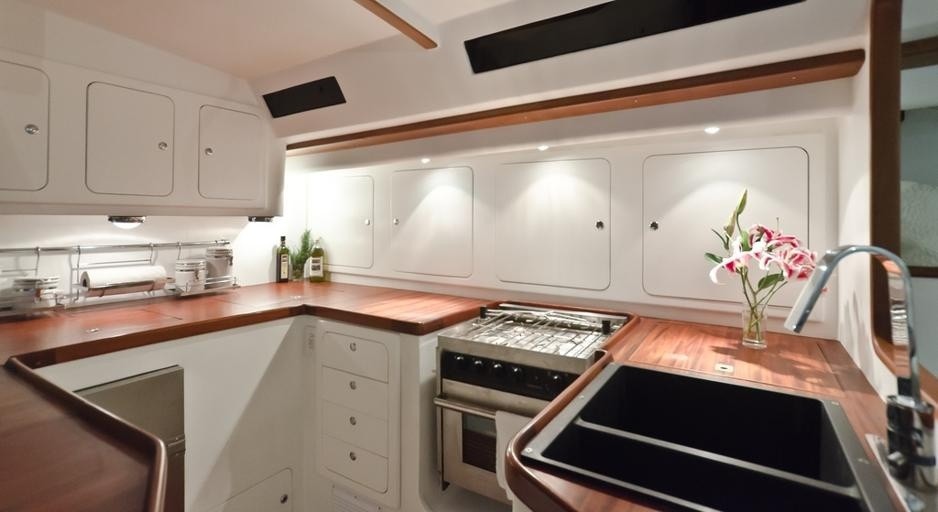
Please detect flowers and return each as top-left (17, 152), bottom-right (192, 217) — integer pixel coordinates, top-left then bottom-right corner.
top-left (705, 192), bottom-right (833, 298)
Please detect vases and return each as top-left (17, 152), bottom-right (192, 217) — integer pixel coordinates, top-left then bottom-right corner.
top-left (737, 268), bottom-right (784, 348)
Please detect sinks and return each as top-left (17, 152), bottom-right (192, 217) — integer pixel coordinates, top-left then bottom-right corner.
top-left (521, 419), bottom-right (898, 512)
top-left (572, 362), bottom-right (885, 496)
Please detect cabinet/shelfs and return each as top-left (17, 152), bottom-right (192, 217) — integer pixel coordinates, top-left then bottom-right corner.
top-left (306, 312), bottom-right (480, 510)
top-left (1, 49), bottom-right (284, 217)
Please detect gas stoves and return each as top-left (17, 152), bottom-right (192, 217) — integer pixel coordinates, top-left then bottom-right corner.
top-left (439, 299), bottom-right (628, 374)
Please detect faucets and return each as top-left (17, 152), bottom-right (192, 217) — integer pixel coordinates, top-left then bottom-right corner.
top-left (782, 246), bottom-right (922, 405)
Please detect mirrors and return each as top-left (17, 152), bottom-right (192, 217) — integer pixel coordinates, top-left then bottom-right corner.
top-left (864, 2), bottom-right (938, 408)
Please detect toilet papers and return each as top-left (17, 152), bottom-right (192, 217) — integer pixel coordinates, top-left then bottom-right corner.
top-left (81, 264), bottom-right (168, 290)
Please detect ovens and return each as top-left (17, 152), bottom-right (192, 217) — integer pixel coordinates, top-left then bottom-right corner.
top-left (436, 350), bottom-right (584, 510)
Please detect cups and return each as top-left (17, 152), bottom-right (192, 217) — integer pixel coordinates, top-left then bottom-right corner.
top-left (742, 301), bottom-right (769, 351)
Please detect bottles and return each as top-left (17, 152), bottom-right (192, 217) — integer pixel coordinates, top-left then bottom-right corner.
top-left (175, 257), bottom-right (208, 294)
top-left (308, 237), bottom-right (326, 285)
top-left (206, 246), bottom-right (234, 278)
top-left (14, 274), bottom-right (59, 312)
top-left (275, 236), bottom-right (291, 283)
